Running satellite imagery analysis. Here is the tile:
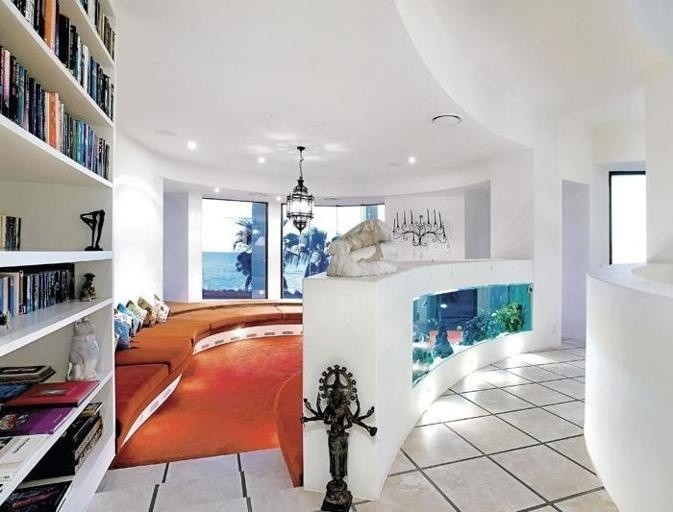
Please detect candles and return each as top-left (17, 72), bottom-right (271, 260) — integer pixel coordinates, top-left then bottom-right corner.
top-left (393, 208), bottom-right (446, 240)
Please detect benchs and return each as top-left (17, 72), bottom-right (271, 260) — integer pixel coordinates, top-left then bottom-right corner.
top-left (115, 306), bottom-right (304, 455)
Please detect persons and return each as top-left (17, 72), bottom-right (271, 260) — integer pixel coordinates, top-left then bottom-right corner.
top-left (298, 387), bottom-right (378, 482)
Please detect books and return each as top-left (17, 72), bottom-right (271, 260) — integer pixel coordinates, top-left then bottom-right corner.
top-left (1, 1), bottom-right (115, 180)
top-left (0, 366), bottom-right (103, 512)
top-left (1, 214), bottom-right (21, 249)
top-left (1, 262), bottom-right (76, 326)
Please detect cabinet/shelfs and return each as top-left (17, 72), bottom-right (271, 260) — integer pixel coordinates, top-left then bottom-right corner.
top-left (2, 0), bottom-right (119, 510)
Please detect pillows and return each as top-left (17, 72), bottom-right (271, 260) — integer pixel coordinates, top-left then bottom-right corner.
top-left (114, 295), bottom-right (170, 348)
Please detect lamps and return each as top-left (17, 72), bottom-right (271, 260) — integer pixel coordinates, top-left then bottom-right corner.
top-left (287, 147), bottom-right (315, 237)
